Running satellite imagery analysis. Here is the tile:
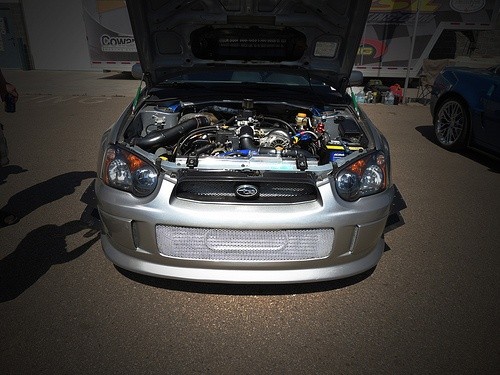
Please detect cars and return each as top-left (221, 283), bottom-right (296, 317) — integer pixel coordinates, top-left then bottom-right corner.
top-left (95, 1), bottom-right (395, 285)
top-left (430, 63), bottom-right (500, 160)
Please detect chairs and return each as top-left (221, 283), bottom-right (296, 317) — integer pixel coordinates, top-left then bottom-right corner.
top-left (416, 58), bottom-right (447, 106)
